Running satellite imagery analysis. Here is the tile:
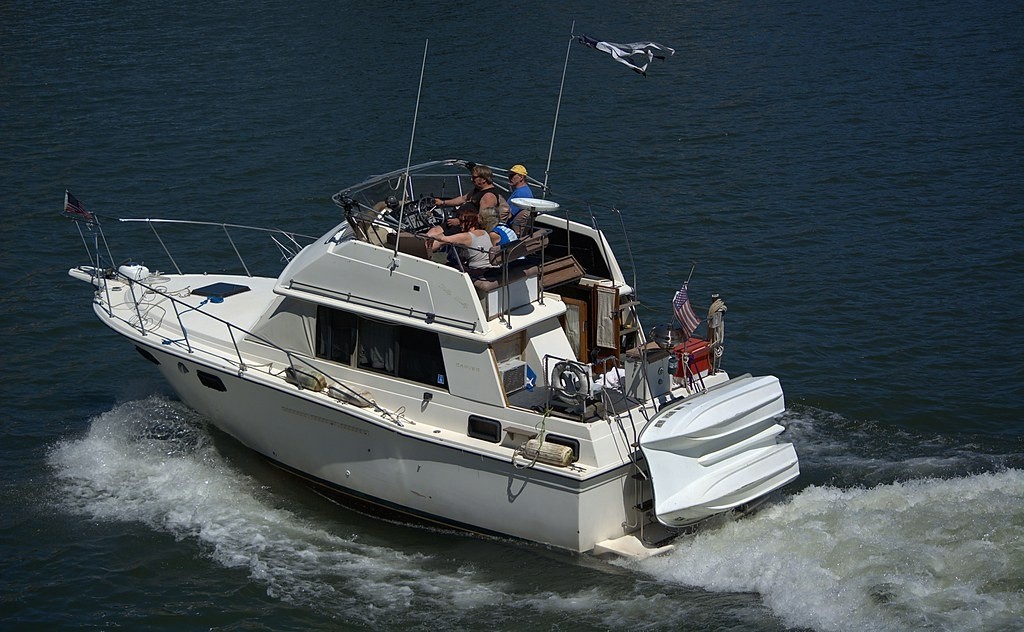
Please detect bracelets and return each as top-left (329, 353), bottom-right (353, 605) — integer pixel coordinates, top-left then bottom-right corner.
top-left (443, 200), bottom-right (445, 205)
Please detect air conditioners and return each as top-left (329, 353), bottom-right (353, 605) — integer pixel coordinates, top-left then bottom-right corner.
top-left (498, 359), bottom-right (528, 395)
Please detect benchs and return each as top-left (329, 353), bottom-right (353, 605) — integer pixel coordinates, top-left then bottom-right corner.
top-left (474, 226), bottom-right (553, 292)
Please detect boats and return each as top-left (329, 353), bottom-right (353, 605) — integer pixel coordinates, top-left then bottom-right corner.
top-left (61, 158), bottom-right (801, 558)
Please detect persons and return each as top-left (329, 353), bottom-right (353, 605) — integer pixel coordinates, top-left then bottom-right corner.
top-left (426, 207), bottom-right (527, 282)
top-left (506, 165), bottom-right (535, 224)
top-left (435, 165), bottom-right (499, 226)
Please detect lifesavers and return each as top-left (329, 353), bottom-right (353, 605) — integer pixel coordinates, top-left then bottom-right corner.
top-left (551, 362), bottom-right (588, 406)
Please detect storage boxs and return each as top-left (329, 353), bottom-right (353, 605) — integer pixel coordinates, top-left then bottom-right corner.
top-left (672, 339), bottom-right (713, 377)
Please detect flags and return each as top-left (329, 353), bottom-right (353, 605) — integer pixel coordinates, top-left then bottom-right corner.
top-left (673, 284), bottom-right (702, 339)
top-left (64, 192), bottom-right (93, 225)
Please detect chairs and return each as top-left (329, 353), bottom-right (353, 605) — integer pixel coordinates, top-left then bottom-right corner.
top-left (512, 208), bottom-right (532, 237)
top-left (397, 233), bottom-right (433, 260)
top-left (496, 203), bottom-right (510, 225)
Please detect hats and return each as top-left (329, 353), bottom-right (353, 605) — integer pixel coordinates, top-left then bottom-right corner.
top-left (510, 165), bottom-right (528, 176)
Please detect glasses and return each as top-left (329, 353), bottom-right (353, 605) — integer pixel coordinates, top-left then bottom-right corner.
top-left (470, 176), bottom-right (479, 181)
top-left (460, 220), bottom-right (465, 223)
